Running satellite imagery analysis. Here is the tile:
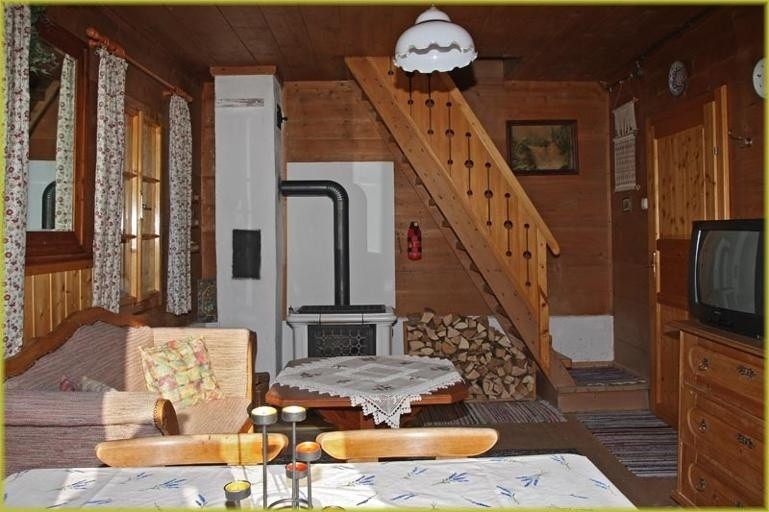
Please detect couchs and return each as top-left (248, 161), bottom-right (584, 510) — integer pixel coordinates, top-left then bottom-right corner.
top-left (3, 306), bottom-right (269, 466)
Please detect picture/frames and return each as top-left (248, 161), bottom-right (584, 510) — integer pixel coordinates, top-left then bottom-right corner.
top-left (505, 119), bottom-right (580, 177)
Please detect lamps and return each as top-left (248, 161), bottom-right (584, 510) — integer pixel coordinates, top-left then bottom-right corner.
top-left (393, 4), bottom-right (478, 76)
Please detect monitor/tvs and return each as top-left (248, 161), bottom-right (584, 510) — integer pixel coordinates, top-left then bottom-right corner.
top-left (687, 218), bottom-right (764, 338)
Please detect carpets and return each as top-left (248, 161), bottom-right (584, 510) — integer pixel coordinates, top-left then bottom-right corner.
top-left (405, 398), bottom-right (567, 428)
top-left (575, 408), bottom-right (680, 478)
top-left (566, 363), bottom-right (648, 387)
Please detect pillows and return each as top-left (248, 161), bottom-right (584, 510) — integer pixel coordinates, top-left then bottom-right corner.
top-left (54, 373), bottom-right (120, 392)
top-left (138, 334), bottom-right (227, 414)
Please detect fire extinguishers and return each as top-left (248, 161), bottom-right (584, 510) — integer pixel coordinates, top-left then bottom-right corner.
top-left (407, 222), bottom-right (422, 260)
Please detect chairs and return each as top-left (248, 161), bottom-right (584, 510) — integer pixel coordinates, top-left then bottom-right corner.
top-left (98, 432), bottom-right (288, 468)
top-left (316, 425), bottom-right (499, 464)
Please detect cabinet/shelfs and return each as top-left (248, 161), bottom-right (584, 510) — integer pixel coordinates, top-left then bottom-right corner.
top-left (665, 320), bottom-right (768, 509)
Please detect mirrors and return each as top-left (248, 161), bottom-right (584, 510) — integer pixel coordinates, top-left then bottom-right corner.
top-left (23, 12), bottom-right (98, 268)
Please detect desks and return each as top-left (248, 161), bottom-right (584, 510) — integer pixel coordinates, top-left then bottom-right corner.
top-left (1, 454), bottom-right (638, 512)
top-left (264, 353), bottom-right (466, 430)
